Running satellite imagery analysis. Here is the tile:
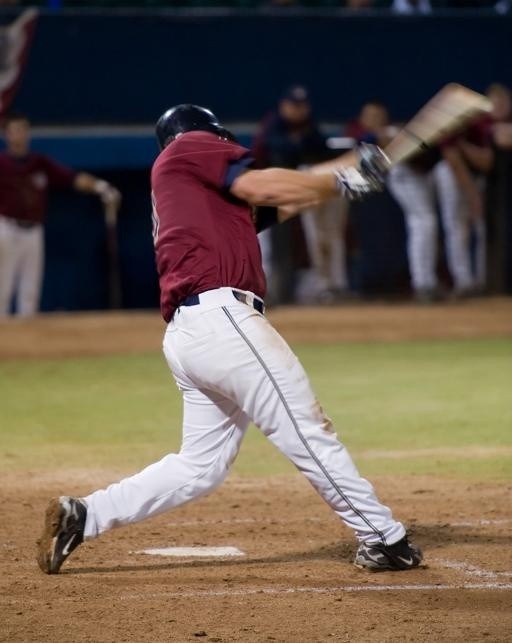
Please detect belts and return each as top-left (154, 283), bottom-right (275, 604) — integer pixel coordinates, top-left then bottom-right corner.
top-left (174, 292), bottom-right (266, 316)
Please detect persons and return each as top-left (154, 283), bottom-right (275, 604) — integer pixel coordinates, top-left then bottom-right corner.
top-left (37, 103), bottom-right (423, 573)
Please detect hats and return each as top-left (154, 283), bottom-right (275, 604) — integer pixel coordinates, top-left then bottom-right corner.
top-left (286, 85), bottom-right (312, 107)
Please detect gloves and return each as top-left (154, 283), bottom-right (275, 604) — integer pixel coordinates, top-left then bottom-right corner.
top-left (337, 142), bottom-right (388, 200)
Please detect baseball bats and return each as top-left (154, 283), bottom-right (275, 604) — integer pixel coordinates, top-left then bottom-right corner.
top-left (384, 84), bottom-right (494, 165)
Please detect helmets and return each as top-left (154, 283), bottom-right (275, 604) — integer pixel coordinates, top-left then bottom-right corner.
top-left (155, 105), bottom-right (237, 144)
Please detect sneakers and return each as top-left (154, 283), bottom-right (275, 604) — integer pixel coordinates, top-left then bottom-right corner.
top-left (351, 537), bottom-right (424, 573)
top-left (37, 490), bottom-right (85, 578)
